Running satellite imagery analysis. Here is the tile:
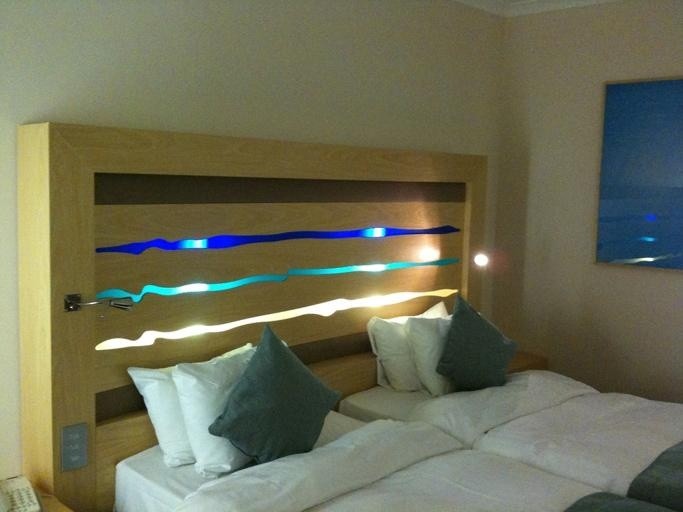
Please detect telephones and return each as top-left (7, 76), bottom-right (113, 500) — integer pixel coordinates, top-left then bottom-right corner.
top-left (0, 474), bottom-right (42, 512)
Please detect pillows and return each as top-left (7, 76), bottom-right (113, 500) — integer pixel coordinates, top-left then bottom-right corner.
top-left (123, 321), bottom-right (340, 477)
top-left (366, 292), bottom-right (518, 396)
